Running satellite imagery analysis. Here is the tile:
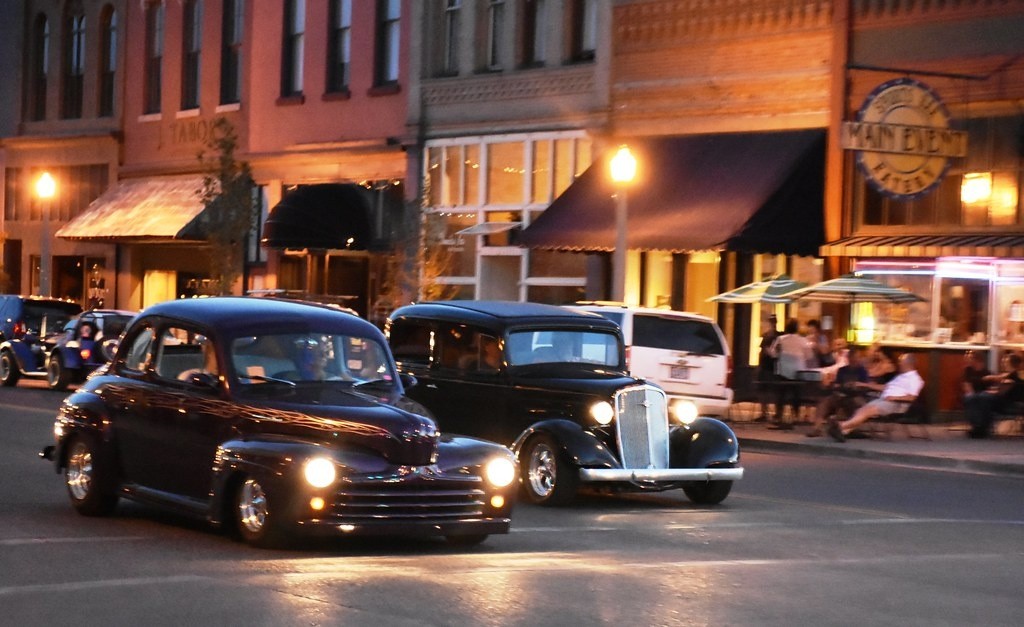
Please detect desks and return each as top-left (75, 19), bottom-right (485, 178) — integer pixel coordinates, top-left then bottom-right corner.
top-left (847, 342), bottom-right (991, 369)
top-left (753, 381), bottom-right (821, 431)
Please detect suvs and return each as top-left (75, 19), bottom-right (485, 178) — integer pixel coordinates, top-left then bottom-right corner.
top-left (572, 300), bottom-right (737, 417)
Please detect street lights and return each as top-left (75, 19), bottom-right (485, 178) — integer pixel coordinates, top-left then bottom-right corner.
top-left (603, 141), bottom-right (644, 301)
top-left (35, 170), bottom-right (58, 297)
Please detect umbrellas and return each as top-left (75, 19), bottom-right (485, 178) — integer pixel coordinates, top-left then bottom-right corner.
top-left (707, 270), bottom-right (812, 330)
top-left (778, 271), bottom-right (929, 328)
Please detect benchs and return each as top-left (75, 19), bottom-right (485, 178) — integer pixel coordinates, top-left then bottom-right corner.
top-left (160, 343), bottom-right (295, 383)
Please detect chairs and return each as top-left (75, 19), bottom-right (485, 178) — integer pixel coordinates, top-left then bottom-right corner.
top-left (858, 387), bottom-right (941, 441)
top-left (728, 365), bottom-right (764, 425)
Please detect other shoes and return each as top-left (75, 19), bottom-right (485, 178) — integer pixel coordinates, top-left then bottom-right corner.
top-left (828, 415), bottom-right (849, 443)
top-left (806, 428), bottom-right (827, 437)
top-left (766, 423), bottom-right (793, 430)
top-left (751, 417), bottom-right (767, 424)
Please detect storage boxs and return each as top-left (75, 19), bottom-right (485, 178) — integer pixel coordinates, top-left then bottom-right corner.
top-left (796, 370), bottom-right (821, 380)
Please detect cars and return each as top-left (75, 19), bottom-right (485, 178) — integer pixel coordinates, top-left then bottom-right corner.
top-left (0, 294), bottom-right (140, 391)
top-left (38, 289), bottom-right (522, 548)
top-left (382, 299), bottom-right (745, 511)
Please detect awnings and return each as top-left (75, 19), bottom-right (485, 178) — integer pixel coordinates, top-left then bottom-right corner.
top-left (261, 183), bottom-right (381, 247)
top-left (54, 170), bottom-right (245, 240)
top-left (515, 127), bottom-right (828, 256)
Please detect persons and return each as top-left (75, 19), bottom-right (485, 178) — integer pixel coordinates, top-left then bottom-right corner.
top-left (178, 340), bottom-right (217, 381)
top-left (807, 343), bottom-right (897, 438)
top-left (471, 341), bottom-right (504, 368)
top-left (752, 315), bottom-right (850, 430)
top-left (962, 350), bottom-right (1024, 438)
top-left (535, 332), bottom-right (585, 362)
top-left (828, 353), bottom-right (924, 442)
top-left (301, 344), bottom-right (335, 379)
